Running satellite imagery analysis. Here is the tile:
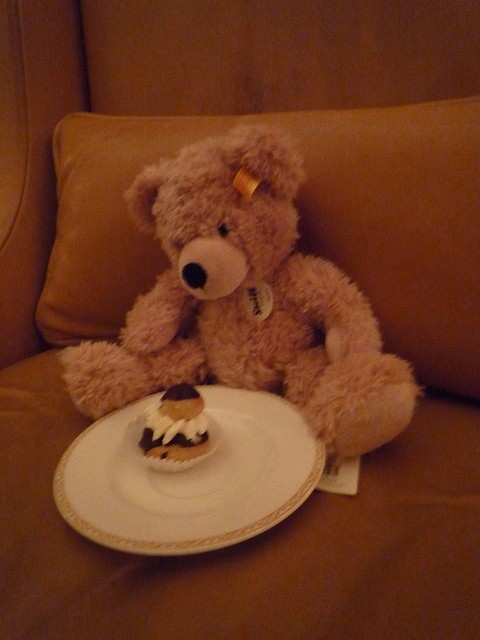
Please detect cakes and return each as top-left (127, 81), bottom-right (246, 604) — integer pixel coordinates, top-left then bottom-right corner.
top-left (136, 385), bottom-right (211, 464)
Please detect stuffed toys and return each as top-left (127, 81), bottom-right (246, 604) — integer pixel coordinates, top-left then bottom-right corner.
top-left (56, 123), bottom-right (424, 463)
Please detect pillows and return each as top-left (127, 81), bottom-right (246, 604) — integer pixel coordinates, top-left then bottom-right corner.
top-left (36, 97), bottom-right (480, 398)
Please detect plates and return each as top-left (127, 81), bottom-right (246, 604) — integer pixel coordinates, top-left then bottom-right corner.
top-left (52, 384), bottom-right (327, 556)
